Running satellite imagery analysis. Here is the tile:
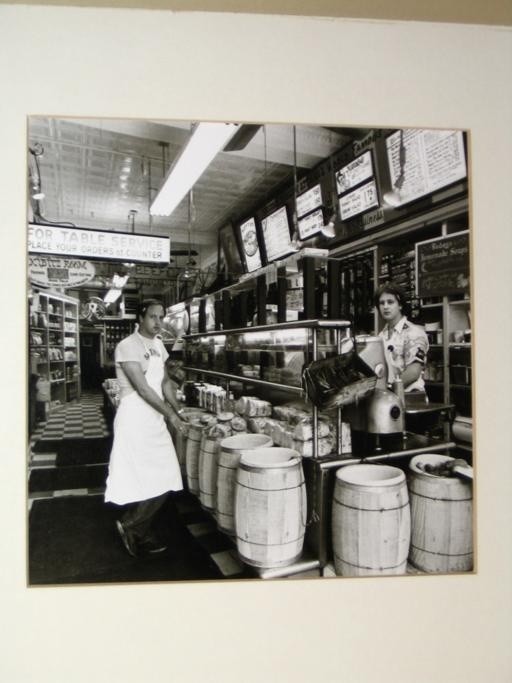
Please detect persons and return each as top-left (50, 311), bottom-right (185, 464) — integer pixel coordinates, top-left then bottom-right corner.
top-left (374, 282), bottom-right (431, 403)
top-left (102, 296), bottom-right (192, 558)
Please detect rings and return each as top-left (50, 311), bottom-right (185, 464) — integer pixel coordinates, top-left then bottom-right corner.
top-left (179, 426), bottom-right (184, 431)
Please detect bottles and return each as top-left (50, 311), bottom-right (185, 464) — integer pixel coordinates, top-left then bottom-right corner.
top-left (392, 373), bottom-right (406, 407)
top-left (437, 329), bottom-right (442, 343)
top-left (185, 380), bottom-right (236, 414)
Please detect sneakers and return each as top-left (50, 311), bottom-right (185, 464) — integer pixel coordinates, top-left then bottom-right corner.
top-left (137, 539), bottom-right (169, 552)
top-left (113, 519), bottom-right (139, 557)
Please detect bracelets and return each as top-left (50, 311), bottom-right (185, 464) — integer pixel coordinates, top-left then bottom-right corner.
top-left (176, 408), bottom-right (185, 414)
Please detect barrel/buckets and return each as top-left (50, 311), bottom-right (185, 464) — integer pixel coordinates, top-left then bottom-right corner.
top-left (330, 463), bottom-right (412, 576)
top-left (175, 423), bottom-right (190, 478)
top-left (197, 433), bottom-right (218, 511)
top-left (408, 454), bottom-right (473, 572)
top-left (185, 424), bottom-right (205, 497)
top-left (233, 447), bottom-right (307, 568)
top-left (216, 435), bottom-right (274, 535)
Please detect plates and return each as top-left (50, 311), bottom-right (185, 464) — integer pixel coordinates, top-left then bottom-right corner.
top-left (244, 231), bottom-right (258, 256)
